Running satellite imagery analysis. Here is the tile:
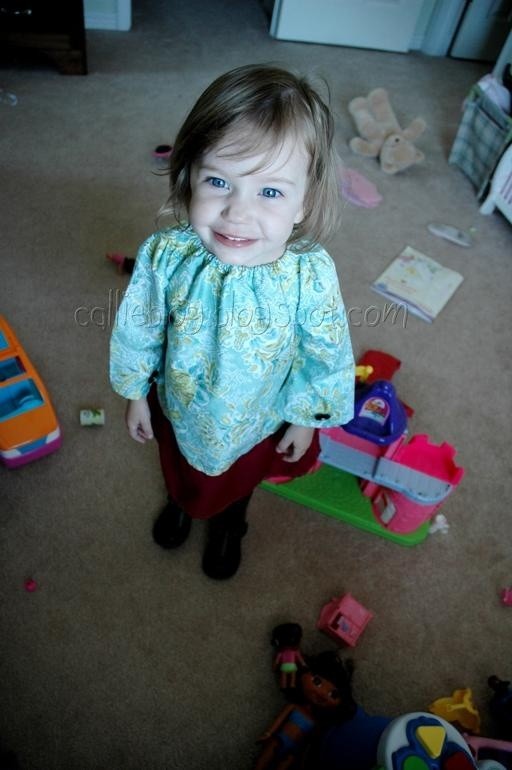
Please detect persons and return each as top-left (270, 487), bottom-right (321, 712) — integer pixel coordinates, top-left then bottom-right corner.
top-left (108, 54), bottom-right (361, 583)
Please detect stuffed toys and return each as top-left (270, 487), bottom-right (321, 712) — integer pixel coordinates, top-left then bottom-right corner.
top-left (246, 589), bottom-right (512, 769)
top-left (0, 314), bottom-right (65, 470)
top-left (256, 349), bottom-right (466, 547)
top-left (348, 87), bottom-right (428, 176)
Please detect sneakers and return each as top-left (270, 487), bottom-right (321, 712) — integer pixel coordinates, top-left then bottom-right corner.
top-left (152, 499), bottom-right (192, 550)
top-left (201, 488), bottom-right (252, 582)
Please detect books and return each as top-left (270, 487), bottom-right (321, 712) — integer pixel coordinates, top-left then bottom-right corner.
top-left (372, 245), bottom-right (465, 325)
